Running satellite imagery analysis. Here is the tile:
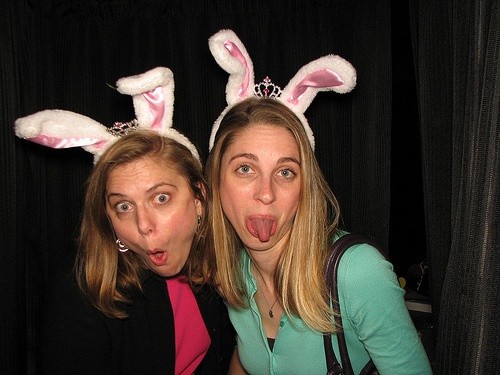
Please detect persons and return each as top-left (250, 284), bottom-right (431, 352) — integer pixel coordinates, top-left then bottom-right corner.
top-left (201, 98), bottom-right (433, 374)
top-left (2, 129), bottom-right (239, 375)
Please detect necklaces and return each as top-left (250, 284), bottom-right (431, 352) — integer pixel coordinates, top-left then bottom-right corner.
top-left (256, 282), bottom-right (281, 318)
